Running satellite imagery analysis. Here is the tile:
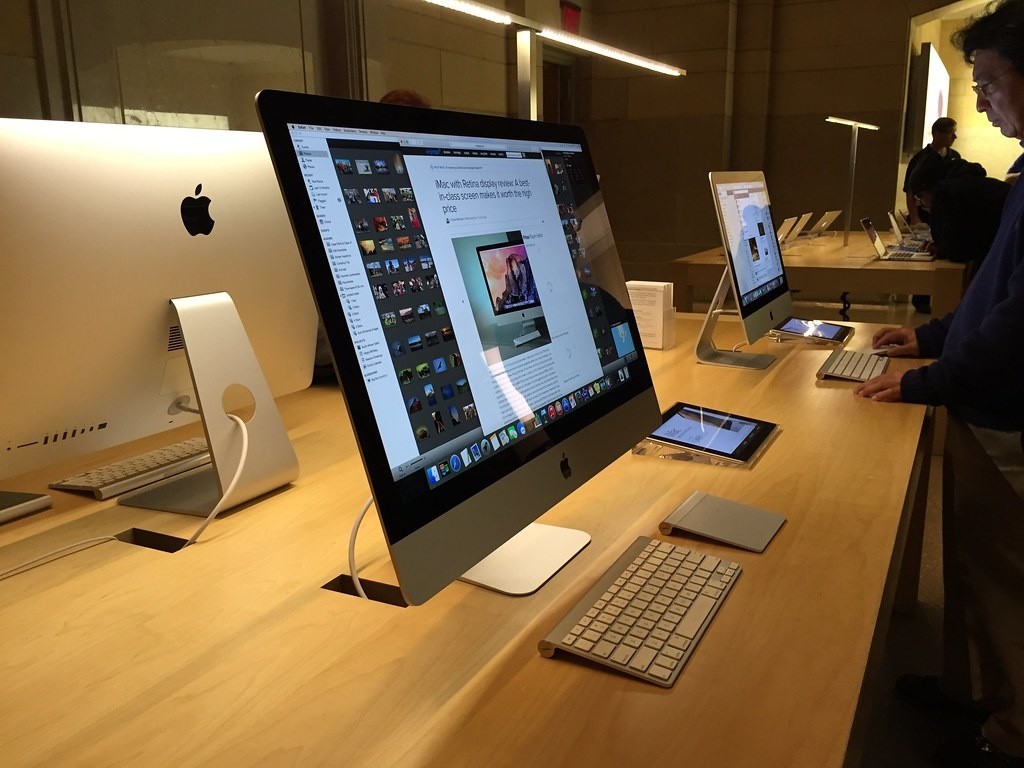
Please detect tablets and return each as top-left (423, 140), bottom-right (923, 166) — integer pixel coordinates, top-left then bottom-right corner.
top-left (773, 317), bottom-right (853, 344)
top-left (645, 401), bottom-right (781, 468)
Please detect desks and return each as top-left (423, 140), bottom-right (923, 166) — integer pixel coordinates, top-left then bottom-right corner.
top-left (0, 382), bottom-right (348, 545)
top-left (674, 231), bottom-right (964, 320)
top-left (0, 310), bottom-right (934, 768)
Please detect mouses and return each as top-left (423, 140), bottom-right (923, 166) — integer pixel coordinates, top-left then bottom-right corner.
top-left (868, 344), bottom-right (900, 356)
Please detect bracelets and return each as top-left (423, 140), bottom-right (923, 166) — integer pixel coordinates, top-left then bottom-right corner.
top-left (926, 240), bottom-right (934, 250)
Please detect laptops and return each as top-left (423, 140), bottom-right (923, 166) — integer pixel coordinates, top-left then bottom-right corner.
top-left (718, 210), bottom-right (841, 256)
top-left (860, 208), bottom-right (936, 261)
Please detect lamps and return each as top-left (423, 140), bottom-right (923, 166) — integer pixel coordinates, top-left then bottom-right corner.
top-left (424, 0), bottom-right (690, 76)
top-left (825, 115), bottom-right (880, 131)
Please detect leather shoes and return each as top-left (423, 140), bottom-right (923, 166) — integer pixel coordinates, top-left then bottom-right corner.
top-left (932, 738), bottom-right (1023, 767)
top-left (894, 673), bottom-right (989, 734)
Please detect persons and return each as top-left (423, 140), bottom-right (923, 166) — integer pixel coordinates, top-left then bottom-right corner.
top-left (376, 89), bottom-right (431, 109)
top-left (854, 0), bottom-right (1024, 768)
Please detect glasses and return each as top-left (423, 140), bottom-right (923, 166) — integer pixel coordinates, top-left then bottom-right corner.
top-left (972, 61), bottom-right (1019, 99)
top-left (913, 193), bottom-right (922, 201)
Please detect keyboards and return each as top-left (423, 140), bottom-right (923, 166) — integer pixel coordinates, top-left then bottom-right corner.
top-left (816, 348), bottom-right (890, 381)
top-left (47, 437), bottom-right (212, 502)
top-left (513, 330), bottom-right (541, 348)
top-left (539, 536), bottom-right (744, 690)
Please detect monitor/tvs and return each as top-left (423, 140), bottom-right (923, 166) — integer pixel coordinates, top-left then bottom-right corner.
top-left (696, 172), bottom-right (794, 371)
top-left (476, 238), bottom-right (545, 336)
top-left (0, 117), bottom-right (319, 518)
top-left (252, 89), bottom-right (662, 606)
